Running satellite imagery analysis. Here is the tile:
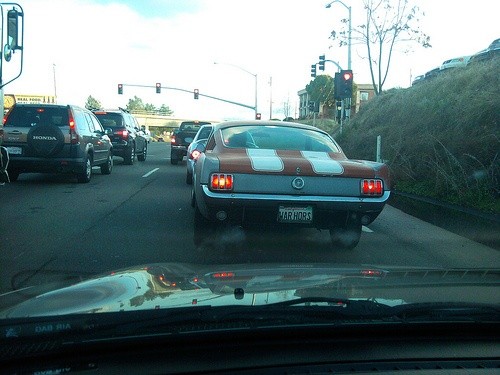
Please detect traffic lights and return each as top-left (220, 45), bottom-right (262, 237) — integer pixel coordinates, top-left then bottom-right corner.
top-left (311, 64), bottom-right (317, 77)
top-left (194, 89), bottom-right (199, 99)
top-left (319, 54), bottom-right (326, 71)
top-left (117, 84), bottom-right (123, 94)
top-left (256, 113), bottom-right (262, 120)
top-left (156, 83), bottom-right (161, 93)
top-left (340, 70), bottom-right (353, 98)
top-left (308, 101), bottom-right (315, 111)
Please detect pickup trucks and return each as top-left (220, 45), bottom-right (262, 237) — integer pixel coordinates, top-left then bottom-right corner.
top-left (170, 121), bottom-right (215, 165)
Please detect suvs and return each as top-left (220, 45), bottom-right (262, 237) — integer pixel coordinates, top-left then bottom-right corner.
top-left (89, 107), bottom-right (150, 165)
top-left (2, 103), bottom-right (115, 184)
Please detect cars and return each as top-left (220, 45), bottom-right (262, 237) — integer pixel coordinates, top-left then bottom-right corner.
top-left (424, 68), bottom-right (440, 79)
top-left (190, 121), bottom-right (392, 250)
top-left (157, 138), bottom-right (164, 142)
top-left (467, 37), bottom-right (500, 67)
top-left (412, 75), bottom-right (425, 86)
top-left (440, 55), bottom-right (469, 71)
top-left (184, 124), bottom-right (238, 185)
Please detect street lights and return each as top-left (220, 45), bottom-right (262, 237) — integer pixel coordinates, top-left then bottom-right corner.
top-left (213, 62), bottom-right (258, 119)
top-left (325, 0), bottom-right (352, 123)
